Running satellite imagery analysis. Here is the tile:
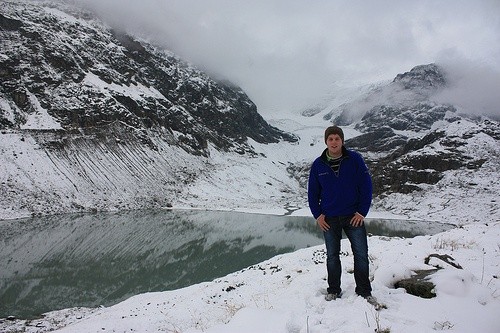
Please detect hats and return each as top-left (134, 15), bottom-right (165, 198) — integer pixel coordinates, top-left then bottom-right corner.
top-left (325, 125), bottom-right (344, 145)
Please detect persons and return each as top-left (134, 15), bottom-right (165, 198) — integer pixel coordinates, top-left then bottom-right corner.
top-left (308, 125), bottom-right (379, 306)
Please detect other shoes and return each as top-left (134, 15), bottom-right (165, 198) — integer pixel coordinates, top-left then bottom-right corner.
top-left (324, 293), bottom-right (337, 301)
top-left (365, 296), bottom-right (381, 309)
